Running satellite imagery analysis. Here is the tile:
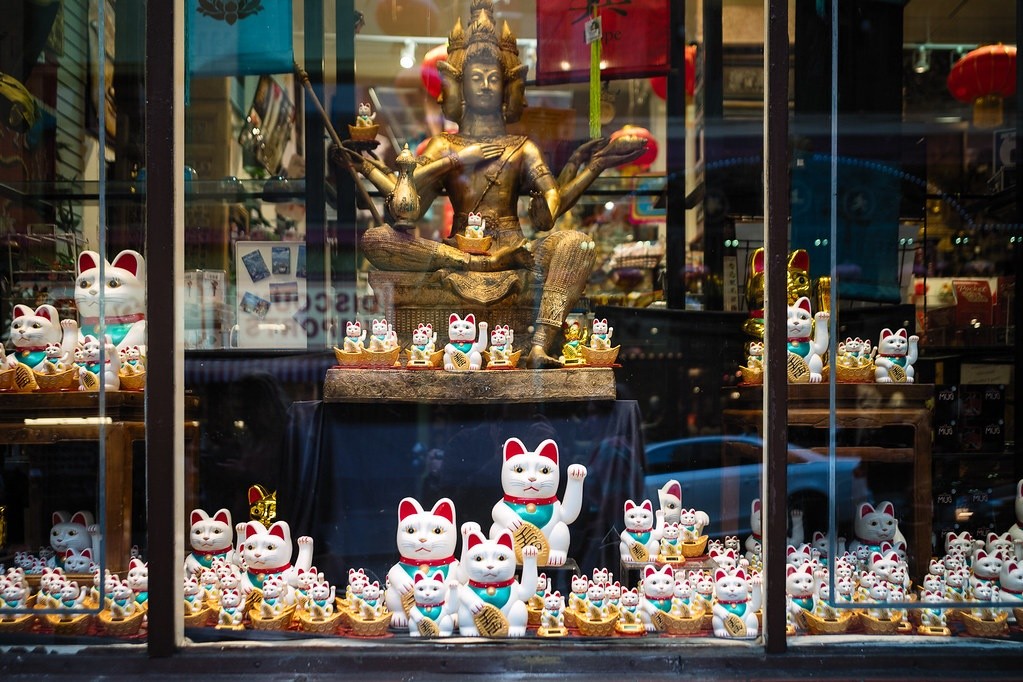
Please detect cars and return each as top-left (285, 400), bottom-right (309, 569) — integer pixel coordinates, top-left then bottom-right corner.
top-left (641, 436), bottom-right (873, 547)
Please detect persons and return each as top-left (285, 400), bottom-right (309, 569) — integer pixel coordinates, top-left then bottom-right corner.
top-left (359, 10), bottom-right (648, 370)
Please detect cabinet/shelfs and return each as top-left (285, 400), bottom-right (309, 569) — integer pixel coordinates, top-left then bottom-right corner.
top-left (0, 0), bottom-right (1023, 682)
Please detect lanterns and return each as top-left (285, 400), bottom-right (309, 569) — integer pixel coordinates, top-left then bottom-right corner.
top-left (609, 125), bottom-right (659, 175)
top-left (420, 41), bottom-right (450, 101)
top-left (946, 39), bottom-right (1016, 127)
top-left (649, 44), bottom-right (697, 108)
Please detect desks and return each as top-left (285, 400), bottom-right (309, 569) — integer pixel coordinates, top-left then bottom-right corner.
top-left (0, 388), bottom-right (201, 588)
top-left (721, 383), bottom-right (942, 582)
